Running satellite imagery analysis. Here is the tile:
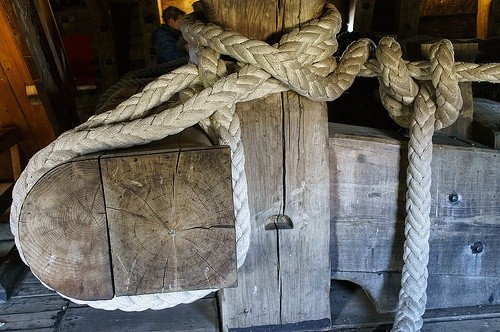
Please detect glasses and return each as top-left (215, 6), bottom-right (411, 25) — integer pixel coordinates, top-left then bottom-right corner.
top-left (176, 19), bottom-right (183, 25)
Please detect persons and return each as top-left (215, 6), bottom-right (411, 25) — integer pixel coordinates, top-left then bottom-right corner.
top-left (150, 6), bottom-right (189, 63)
